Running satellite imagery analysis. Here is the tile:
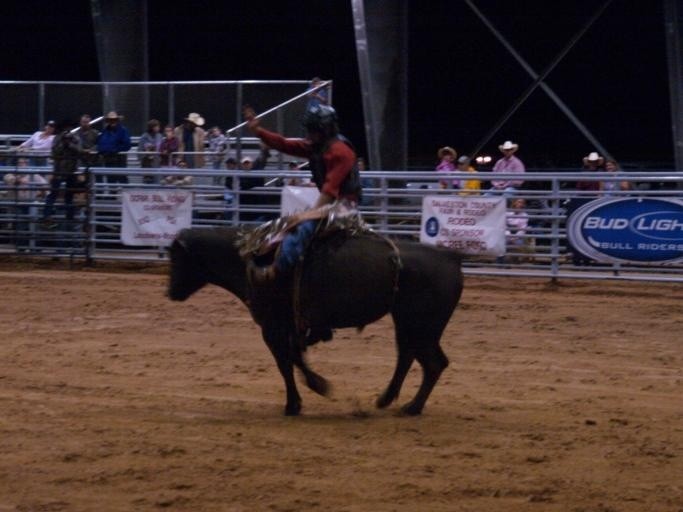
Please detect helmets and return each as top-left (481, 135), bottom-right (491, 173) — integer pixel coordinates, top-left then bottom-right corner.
top-left (301, 105), bottom-right (338, 142)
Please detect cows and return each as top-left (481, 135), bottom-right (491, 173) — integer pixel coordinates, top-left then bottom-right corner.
top-left (165, 223), bottom-right (466, 416)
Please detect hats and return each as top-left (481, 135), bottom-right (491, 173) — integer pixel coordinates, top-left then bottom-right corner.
top-left (438, 146), bottom-right (457, 162)
top-left (498, 141), bottom-right (519, 154)
top-left (103, 111), bottom-right (125, 123)
top-left (47, 120), bottom-right (57, 128)
top-left (583, 152), bottom-right (604, 166)
top-left (457, 155), bottom-right (469, 164)
top-left (184, 113), bottom-right (205, 126)
top-left (241, 157), bottom-right (254, 163)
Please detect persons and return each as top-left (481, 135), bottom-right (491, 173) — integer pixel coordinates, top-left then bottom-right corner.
top-left (305, 78), bottom-right (326, 110)
top-left (436, 141), bottom-right (631, 269)
top-left (244, 103), bottom-right (361, 318)
top-left (1, 109), bottom-right (314, 251)
